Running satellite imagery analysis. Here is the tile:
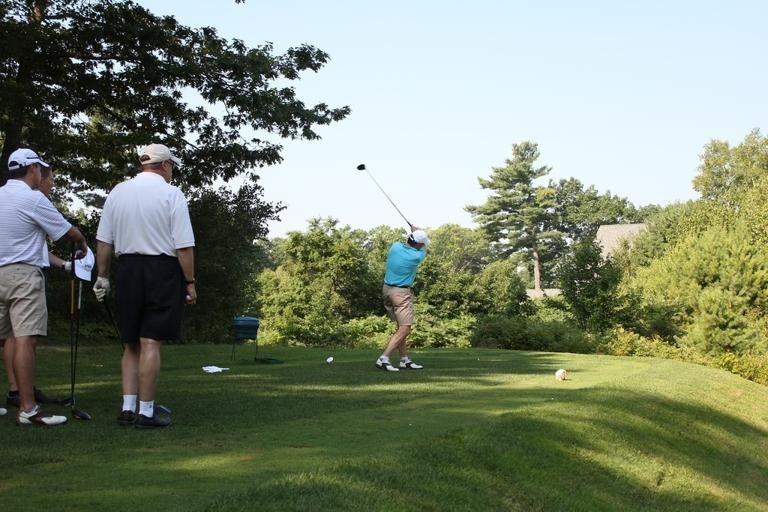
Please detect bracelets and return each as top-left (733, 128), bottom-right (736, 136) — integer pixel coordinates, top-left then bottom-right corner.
top-left (61, 262), bottom-right (65, 271)
top-left (187, 277), bottom-right (195, 284)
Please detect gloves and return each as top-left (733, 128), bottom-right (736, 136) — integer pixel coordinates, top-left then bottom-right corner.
top-left (93, 277), bottom-right (110, 304)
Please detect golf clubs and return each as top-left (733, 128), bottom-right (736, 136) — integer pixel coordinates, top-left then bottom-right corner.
top-left (97, 286), bottom-right (172, 415)
top-left (62, 243), bottom-right (91, 419)
top-left (357, 164), bottom-right (412, 227)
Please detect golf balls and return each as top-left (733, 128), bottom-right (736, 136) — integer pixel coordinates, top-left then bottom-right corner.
top-left (326, 356), bottom-right (334, 365)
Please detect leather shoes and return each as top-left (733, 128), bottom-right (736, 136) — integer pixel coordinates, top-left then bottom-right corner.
top-left (118, 410), bottom-right (171, 429)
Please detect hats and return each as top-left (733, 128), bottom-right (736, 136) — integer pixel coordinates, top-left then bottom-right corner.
top-left (7, 148), bottom-right (49, 170)
top-left (409, 230), bottom-right (431, 245)
top-left (74, 246), bottom-right (94, 282)
top-left (139, 144), bottom-right (180, 164)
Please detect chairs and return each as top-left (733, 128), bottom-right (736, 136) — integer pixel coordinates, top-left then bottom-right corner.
top-left (230, 314), bottom-right (262, 361)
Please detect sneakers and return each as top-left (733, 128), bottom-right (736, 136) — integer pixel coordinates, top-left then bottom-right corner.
top-left (399, 359), bottom-right (422, 369)
top-left (374, 358), bottom-right (399, 372)
top-left (16, 405), bottom-right (67, 426)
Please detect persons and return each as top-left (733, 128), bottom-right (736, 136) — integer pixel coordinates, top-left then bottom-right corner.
top-left (92, 143), bottom-right (197, 428)
top-left (375, 224), bottom-right (431, 372)
top-left (3, 166), bottom-right (73, 408)
top-left (0, 148), bottom-right (87, 427)
